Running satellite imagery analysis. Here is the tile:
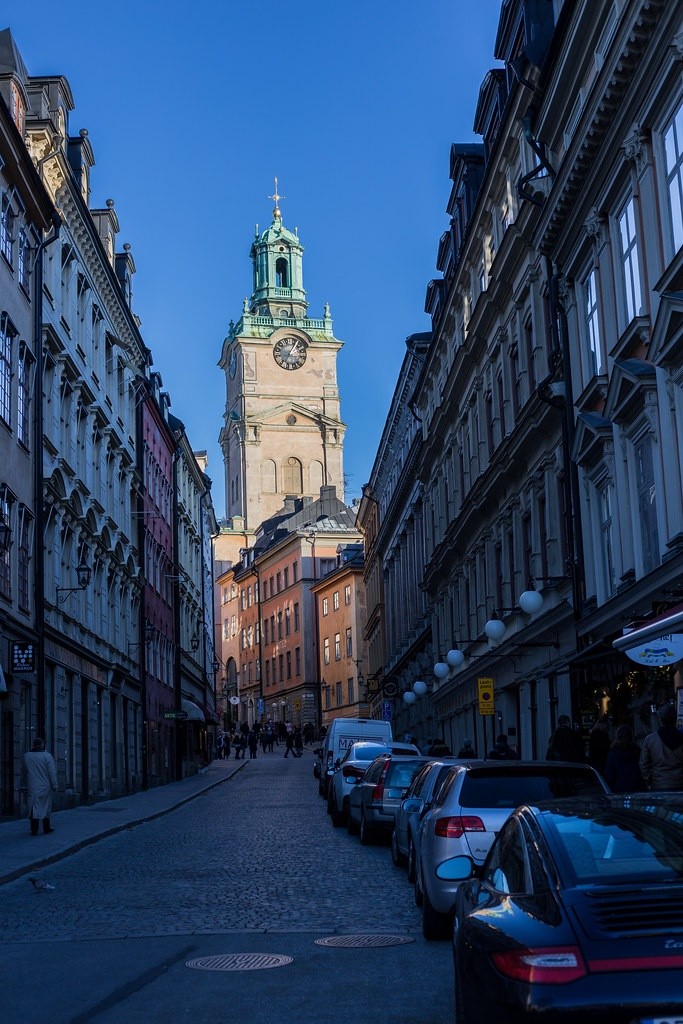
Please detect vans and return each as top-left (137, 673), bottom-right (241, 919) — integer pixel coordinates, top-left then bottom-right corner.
top-left (312, 716), bottom-right (394, 801)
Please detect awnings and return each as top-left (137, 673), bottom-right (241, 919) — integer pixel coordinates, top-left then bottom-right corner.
top-left (533, 630), bottom-right (621, 681)
top-left (119, 356), bottom-right (148, 414)
top-left (106, 331), bottom-right (135, 372)
top-left (62, 241), bottom-right (92, 319)
top-left (612, 603), bottom-right (683, 652)
top-left (182, 699), bottom-right (205, 723)
top-left (199, 704), bottom-right (220, 725)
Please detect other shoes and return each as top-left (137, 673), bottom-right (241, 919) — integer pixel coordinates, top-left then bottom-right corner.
top-left (45, 827), bottom-right (55, 834)
top-left (30, 830), bottom-right (38, 836)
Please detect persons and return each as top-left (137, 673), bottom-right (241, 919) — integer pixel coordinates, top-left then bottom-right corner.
top-left (639, 704), bottom-right (683, 793)
top-left (411, 736), bottom-right (451, 756)
top-left (486, 734), bottom-right (521, 760)
top-left (216, 729), bottom-right (258, 760)
top-left (604, 725), bottom-right (643, 794)
top-left (457, 738), bottom-right (478, 758)
top-left (300, 720), bottom-right (327, 744)
top-left (545, 714), bottom-right (584, 761)
top-left (241, 720), bottom-right (292, 754)
top-left (23, 738), bottom-right (58, 837)
top-left (588, 719), bottom-right (613, 776)
top-left (283, 730), bottom-right (304, 758)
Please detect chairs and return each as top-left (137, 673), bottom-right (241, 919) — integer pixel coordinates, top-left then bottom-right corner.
top-left (557, 831), bottom-right (600, 872)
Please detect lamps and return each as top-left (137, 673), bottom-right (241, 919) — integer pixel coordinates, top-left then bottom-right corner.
top-left (216, 684), bottom-right (231, 702)
top-left (271, 699), bottom-right (279, 707)
top-left (185, 631), bottom-right (199, 654)
top-left (0, 508), bottom-right (14, 557)
top-left (433, 653), bottom-right (449, 677)
top-left (56, 555), bottom-right (92, 603)
top-left (321, 677), bottom-right (335, 697)
top-left (357, 671), bottom-right (368, 686)
top-left (413, 673), bottom-right (435, 695)
top-left (517, 574), bottom-right (565, 615)
top-left (484, 606), bottom-right (521, 640)
top-left (446, 637), bottom-right (488, 667)
top-left (206, 655), bottom-right (220, 675)
top-left (127, 618), bottom-right (157, 659)
top-left (279, 697), bottom-right (287, 706)
top-left (403, 682), bottom-right (415, 704)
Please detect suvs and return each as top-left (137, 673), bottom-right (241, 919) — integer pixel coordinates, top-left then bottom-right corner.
top-left (326, 738), bottom-right (424, 828)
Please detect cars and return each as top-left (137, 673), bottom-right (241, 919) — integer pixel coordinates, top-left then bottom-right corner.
top-left (398, 757), bottom-right (614, 943)
top-left (344, 751), bottom-right (441, 847)
top-left (385, 759), bottom-right (501, 884)
top-left (434, 790), bottom-right (683, 1024)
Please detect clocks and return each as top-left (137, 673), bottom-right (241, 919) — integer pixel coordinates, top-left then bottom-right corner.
top-left (273, 337), bottom-right (307, 371)
top-left (228, 351), bottom-right (237, 381)
